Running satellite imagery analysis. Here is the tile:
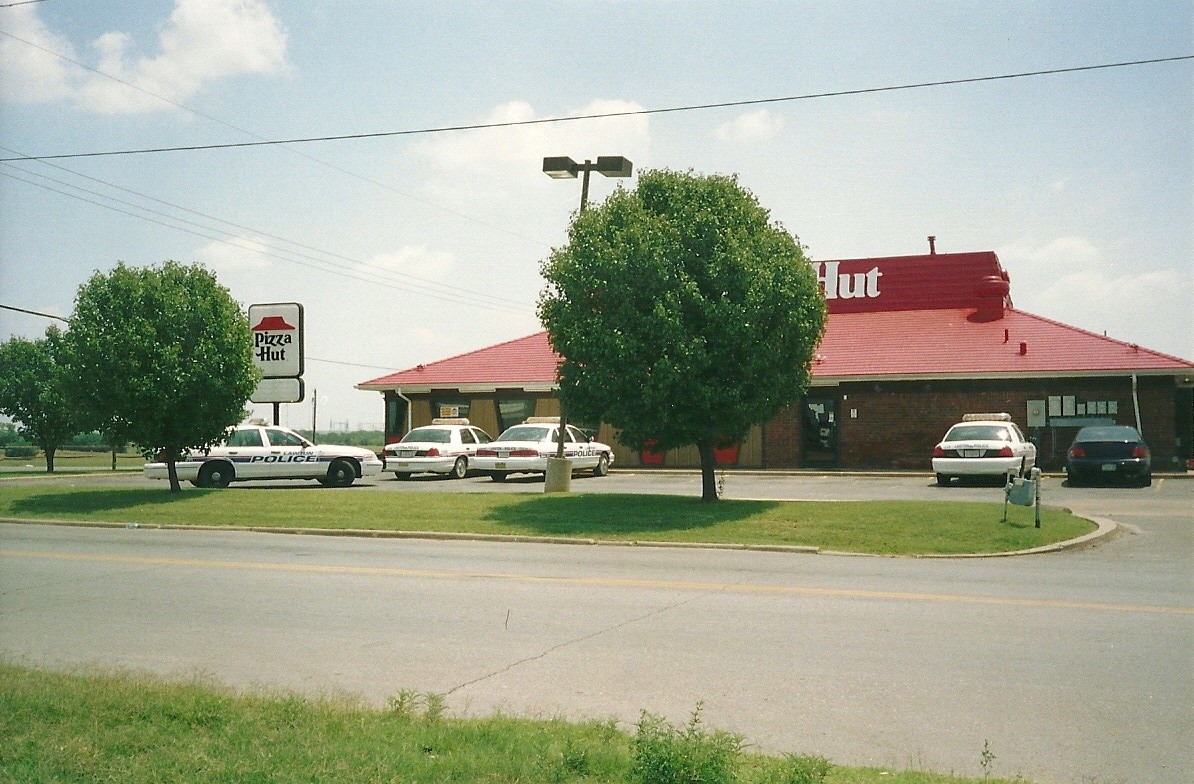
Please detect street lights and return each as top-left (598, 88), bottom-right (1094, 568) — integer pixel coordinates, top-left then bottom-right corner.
top-left (542, 154), bottom-right (634, 490)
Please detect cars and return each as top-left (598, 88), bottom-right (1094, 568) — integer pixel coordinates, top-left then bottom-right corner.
top-left (376, 451), bottom-right (386, 469)
top-left (931, 413), bottom-right (1038, 484)
top-left (475, 416), bottom-right (615, 481)
top-left (381, 418), bottom-right (508, 483)
top-left (1066, 424), bottom-right (1152, 487)
top-left (142, 417), bottom-right (384, 488)
